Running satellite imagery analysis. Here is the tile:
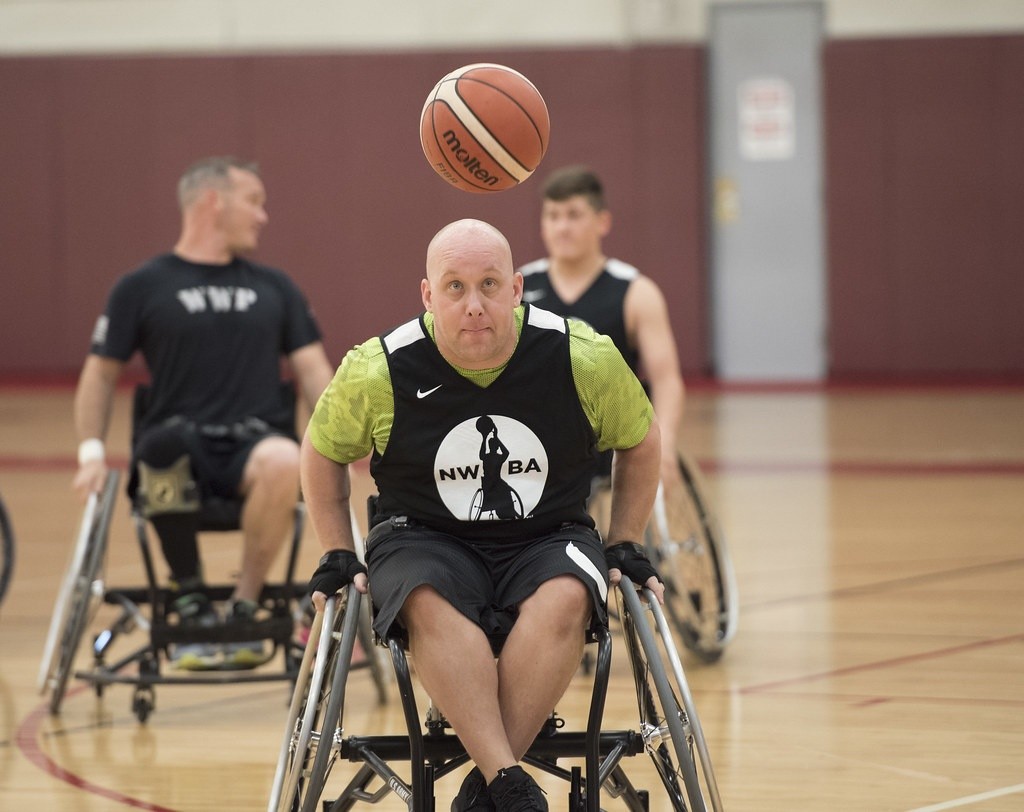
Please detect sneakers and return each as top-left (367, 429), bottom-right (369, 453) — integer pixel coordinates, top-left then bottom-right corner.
top-left (221, 597), bottom-right (263, 669)
top-left (487, 766), bottom-right (549, 812)
top-left (451, 766), bottom-right (496, 812)
top-left (170, 593), bottom-right (224, 670)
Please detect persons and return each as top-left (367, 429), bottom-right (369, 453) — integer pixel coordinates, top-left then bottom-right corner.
top-left (70, 157), bottom-right (339, 667)
top-left (515, 171), bottom-right (682, 570)
top-left (298, 217), bottom-right (666, 812)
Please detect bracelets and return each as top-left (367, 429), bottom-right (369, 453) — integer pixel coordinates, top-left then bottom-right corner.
top-left (77, 437), bottom-right (106, 464)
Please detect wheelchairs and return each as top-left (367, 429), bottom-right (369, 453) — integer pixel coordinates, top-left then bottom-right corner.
top-left (264, 496), bottom-right (722, 812)
top-left (577, 455), bottom-right (738, 671)
top-left (27, 381), bottom-right (390, 718)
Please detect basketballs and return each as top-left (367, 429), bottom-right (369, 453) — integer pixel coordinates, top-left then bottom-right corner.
top-left (419, 62), bottom-right (549, 198)
